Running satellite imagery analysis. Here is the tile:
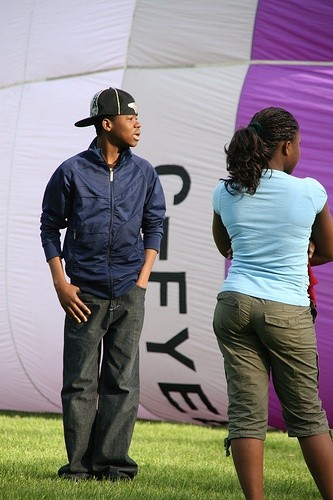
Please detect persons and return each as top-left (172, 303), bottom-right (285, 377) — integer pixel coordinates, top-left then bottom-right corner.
top-left (41, 89), bottom-right (165, 480)
top-left (212, 103), bottom-right (333, 498)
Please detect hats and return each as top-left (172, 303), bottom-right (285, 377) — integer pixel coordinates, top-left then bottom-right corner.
top-left (74, 87), bottom-right (138, 127)
